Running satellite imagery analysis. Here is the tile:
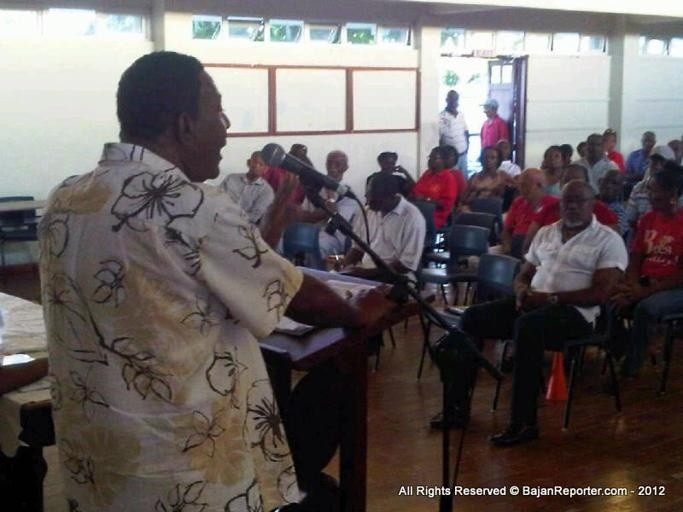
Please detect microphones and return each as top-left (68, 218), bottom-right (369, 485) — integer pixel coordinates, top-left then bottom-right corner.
top-left (260, 144), bottom-right (351, 200)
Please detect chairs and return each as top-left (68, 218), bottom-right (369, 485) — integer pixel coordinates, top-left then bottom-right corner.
top-left (280, 223), bottom-right (321, 269)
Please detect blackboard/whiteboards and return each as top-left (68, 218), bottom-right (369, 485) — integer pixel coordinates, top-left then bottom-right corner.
top-left (272, 64), bottom-right (350, 137)
top-left (350, 64), bottom-right (420, 136)
top-left (201, 62), bottom-right (272, 136)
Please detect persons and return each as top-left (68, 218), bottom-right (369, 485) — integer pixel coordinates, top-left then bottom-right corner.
top-left (36, 50), bottom-right (367, 512)
top-left (0, 342), bottom-right (50, 398)
top-left (215, 89), bottom-right (683, 448)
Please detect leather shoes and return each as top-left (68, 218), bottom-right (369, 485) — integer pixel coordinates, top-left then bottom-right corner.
top-left (431, 411), bottom-right (470, 428)
top-left (491, 425), bottom-right (539, 445)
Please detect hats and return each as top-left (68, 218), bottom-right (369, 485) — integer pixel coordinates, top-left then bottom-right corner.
top-left (480, 99), bottom-right (498, 106)
top-left (648, 145), bottom-right (675, 162)
